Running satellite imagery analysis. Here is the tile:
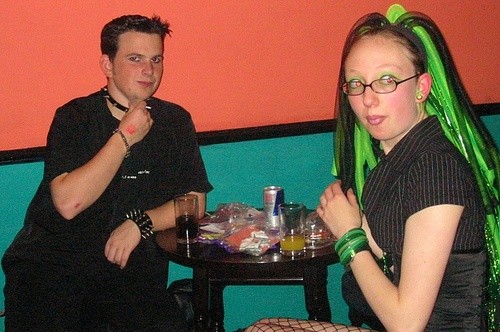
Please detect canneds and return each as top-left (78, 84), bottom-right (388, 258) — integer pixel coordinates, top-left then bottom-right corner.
top-left (263, 186), bottom-right (285, 230)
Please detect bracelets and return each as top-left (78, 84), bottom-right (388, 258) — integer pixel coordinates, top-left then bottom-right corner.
top-left (125, 208), bottom-right (154, 241)
top-left (111, 129), bottom-right (131, 159)
top-left (335, 227), bottom-right (369, 265)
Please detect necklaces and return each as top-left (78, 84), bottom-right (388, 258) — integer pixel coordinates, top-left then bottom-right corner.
top-left (102, 86), bottom-right (130, 114)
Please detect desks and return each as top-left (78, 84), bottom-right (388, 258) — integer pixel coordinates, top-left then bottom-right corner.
top-left (154, 207), bottom-right (340, 332)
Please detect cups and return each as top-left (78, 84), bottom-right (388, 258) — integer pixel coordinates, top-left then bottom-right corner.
top-left (173, 194), bottom-right (198, 244)
top-left (278, 203), bottom-right (305, 256)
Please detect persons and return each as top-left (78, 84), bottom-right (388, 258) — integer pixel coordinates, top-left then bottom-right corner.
top-left (2, 14), bottom-right (214, 332)
top-left (239, 4), bottom-right (500, 332)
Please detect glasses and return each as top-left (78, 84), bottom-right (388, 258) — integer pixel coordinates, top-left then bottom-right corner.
top-left (340, 73), bottom-right (421, 96)
